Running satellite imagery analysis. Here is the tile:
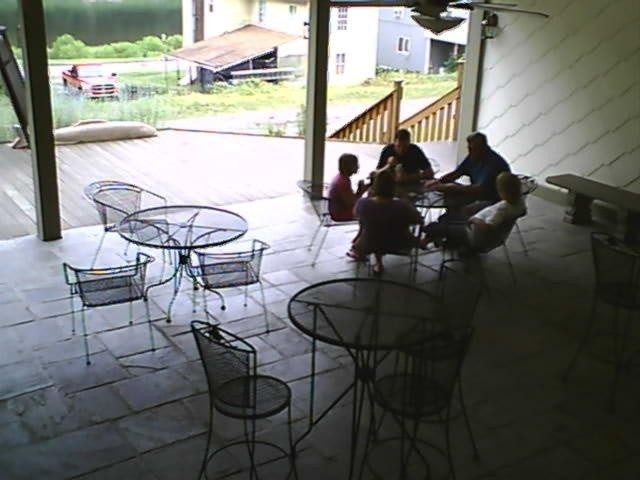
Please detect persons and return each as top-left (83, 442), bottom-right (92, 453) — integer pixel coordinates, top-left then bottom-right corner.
top-left (435, 133), bottom-right (512, 221)
top-left (352, 172), bottom-right (421, 274)
top-left (375, 130), bottom-right (434, 184)
top-left (328, 154), bottom-right (368, 262)
top-left (419, 171), bottom-right (527, 251)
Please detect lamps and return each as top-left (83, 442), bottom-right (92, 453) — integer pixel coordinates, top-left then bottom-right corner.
top-left (480, 8), bottom-right (498, 40)
top-left (410, 9), bottom-right (466, 35)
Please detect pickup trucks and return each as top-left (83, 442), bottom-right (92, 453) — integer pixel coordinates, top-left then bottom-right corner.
top-left (62, 60), bottom-right (120, 99)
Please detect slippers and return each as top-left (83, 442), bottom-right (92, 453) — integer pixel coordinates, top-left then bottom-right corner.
top-left (347, 249), bottom-right (369, 263)
top-left (417, 238), bottom-right (428, 251)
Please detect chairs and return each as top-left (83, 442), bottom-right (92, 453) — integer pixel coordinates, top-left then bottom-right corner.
top-left (560, 232), bottom-right (640, 415)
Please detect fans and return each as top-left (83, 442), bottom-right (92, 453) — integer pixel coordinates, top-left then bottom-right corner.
top-left (326, 0), bottom-right (549, 36)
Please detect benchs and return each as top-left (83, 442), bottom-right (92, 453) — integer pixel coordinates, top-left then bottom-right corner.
top-left (546, 173), bottom-right (640, 244)
